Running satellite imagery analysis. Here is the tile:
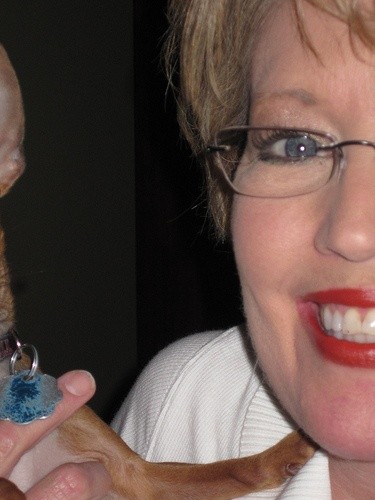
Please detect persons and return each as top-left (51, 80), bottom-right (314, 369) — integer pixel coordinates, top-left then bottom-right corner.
top-left (0, 0), bottom-right (375, 500)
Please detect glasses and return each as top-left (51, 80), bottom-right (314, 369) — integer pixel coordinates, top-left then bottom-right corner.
top-left (206, 127), bottom-right (375, 198)
